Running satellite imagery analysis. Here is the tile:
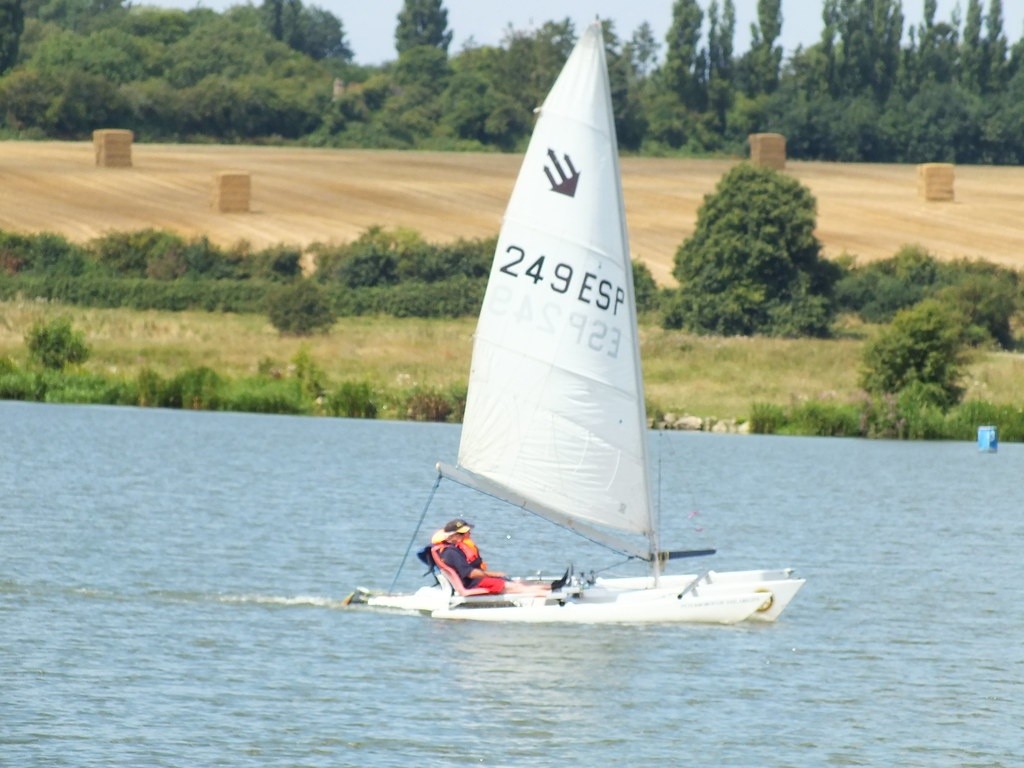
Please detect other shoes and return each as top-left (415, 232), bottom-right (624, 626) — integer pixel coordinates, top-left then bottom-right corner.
top-left (551, 565), bottom-right (573, 591)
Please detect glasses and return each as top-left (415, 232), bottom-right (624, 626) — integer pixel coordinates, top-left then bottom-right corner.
top-left (457, 533), bottom-right (464, 535)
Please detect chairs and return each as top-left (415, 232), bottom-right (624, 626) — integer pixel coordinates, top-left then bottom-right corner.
top-left (431, 549), bottom-right (489, 596)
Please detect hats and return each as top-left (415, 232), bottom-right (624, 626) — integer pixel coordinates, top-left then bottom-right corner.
top-left (444, 519), bottom-right (470, 533)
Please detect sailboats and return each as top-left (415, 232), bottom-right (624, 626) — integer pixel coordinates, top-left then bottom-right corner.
top-left (344, 11), bottom-right (806, 623)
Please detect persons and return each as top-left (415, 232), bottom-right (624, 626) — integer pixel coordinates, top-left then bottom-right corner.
top-left (432, 519), bottom-right (568, 595)
